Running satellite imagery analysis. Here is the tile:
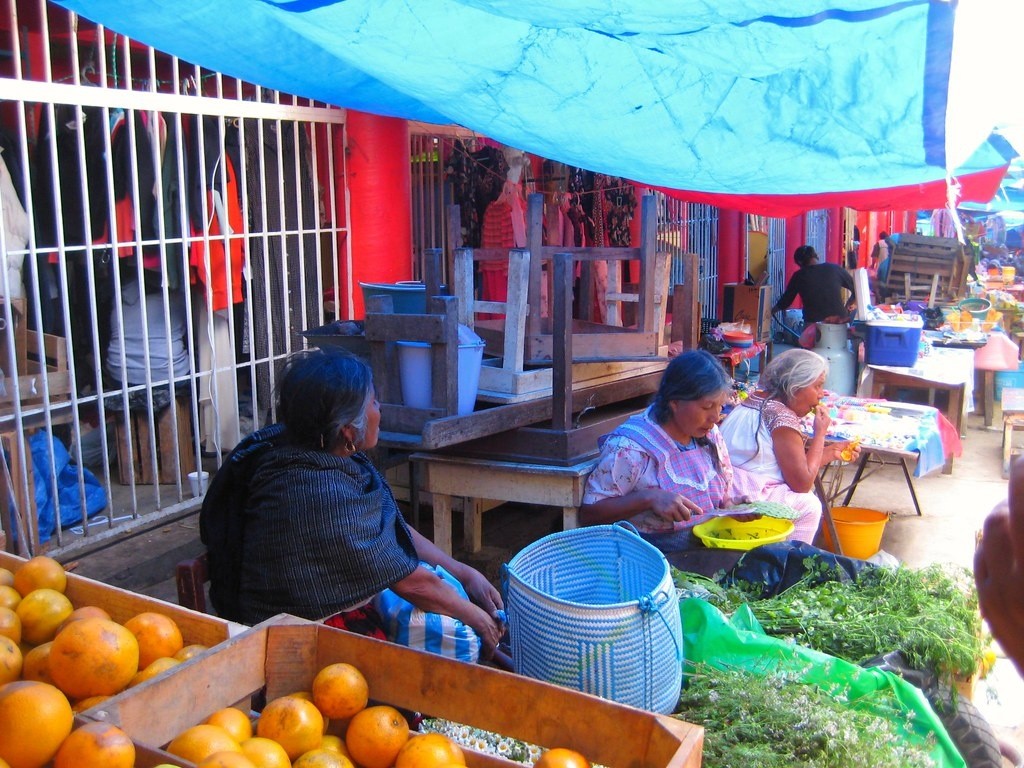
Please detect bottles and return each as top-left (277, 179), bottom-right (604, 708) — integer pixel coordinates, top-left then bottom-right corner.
top-left (867, 304), bottom-right (890, 322)
top-left (889, 305), bottom-right (902, 322)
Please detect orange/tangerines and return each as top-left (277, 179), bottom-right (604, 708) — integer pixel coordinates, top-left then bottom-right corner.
top-left (533, 748), bottom-right (591, 768)
top-left (0, 556), bottom-right (466, 768)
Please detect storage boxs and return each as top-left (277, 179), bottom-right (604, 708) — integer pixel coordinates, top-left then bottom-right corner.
top-left (855, 267), bottom-right (924, 367)
top-left (723, 282), bottom-right (773, 341)
top-left (68, 613), bottom-right (705, 768)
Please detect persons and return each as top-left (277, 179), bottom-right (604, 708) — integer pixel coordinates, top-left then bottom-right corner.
top-left (200, 344), bottom-right (506, 667)
top-left (870, 231), bottom-right (888, 267)
top-left (772, 246), bottom-right (855, 329)
top-left (579, 350), bottom-right (763, 578)
top-left (718, 349), bottom-right (862, 544)
top-left (106, 275), bottom-right (189, 388)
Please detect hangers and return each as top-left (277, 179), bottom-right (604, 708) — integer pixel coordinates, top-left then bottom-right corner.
top-left (79, 65), bottom-right (246, 130)
top-left (493, 180), bottom-right (581, 212)
top-left (466, 131), bottom-right (490, 154)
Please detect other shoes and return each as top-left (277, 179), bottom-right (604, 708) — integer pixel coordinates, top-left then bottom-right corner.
top-left (201, 445), bottom-right (227, 458)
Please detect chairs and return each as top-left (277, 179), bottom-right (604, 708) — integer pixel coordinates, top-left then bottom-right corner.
top-left (174, 547), bottom-right (265, 712)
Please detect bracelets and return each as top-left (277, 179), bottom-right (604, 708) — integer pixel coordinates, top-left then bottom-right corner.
top-left (496, 643), bottom-right (499, 647)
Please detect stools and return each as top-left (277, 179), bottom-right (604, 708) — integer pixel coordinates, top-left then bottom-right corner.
top-left (420, 187), bottom-right (673, 404)
top-left (1000, 386), bottom-right (1024, 479)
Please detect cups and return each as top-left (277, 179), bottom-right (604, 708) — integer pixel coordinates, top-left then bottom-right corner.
top-left (188, 472), bottom-right (210, 498)
top-left (1002, 267), bottom-right (1016, 288)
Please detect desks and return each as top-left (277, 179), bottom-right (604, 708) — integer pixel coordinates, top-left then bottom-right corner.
top-left (930, 317), bottom-right (1021, 427)
top-left (406, 448), bottom-right (602, 562)
top-left (869, 349), bottom-right (975, 452)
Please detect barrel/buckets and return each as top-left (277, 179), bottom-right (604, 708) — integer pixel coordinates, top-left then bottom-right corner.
top-left (995, 362), bottom-right (1024, 401)
top-left (692, 516), bottom-right (795, 552)
top-left (821, 508), bottom-right (897, 561)
top-left (958, 298), bottom-right (991, 320)
top-left (394, 341), bottom-right (486, 416)
top-left (360, 282), bottom-right (449, 314)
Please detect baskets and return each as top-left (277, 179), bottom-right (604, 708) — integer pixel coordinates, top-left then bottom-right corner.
top-left (500, 520), bottom-right (684, 715)
top-left (701, 317), bottom-right (721, 334)
